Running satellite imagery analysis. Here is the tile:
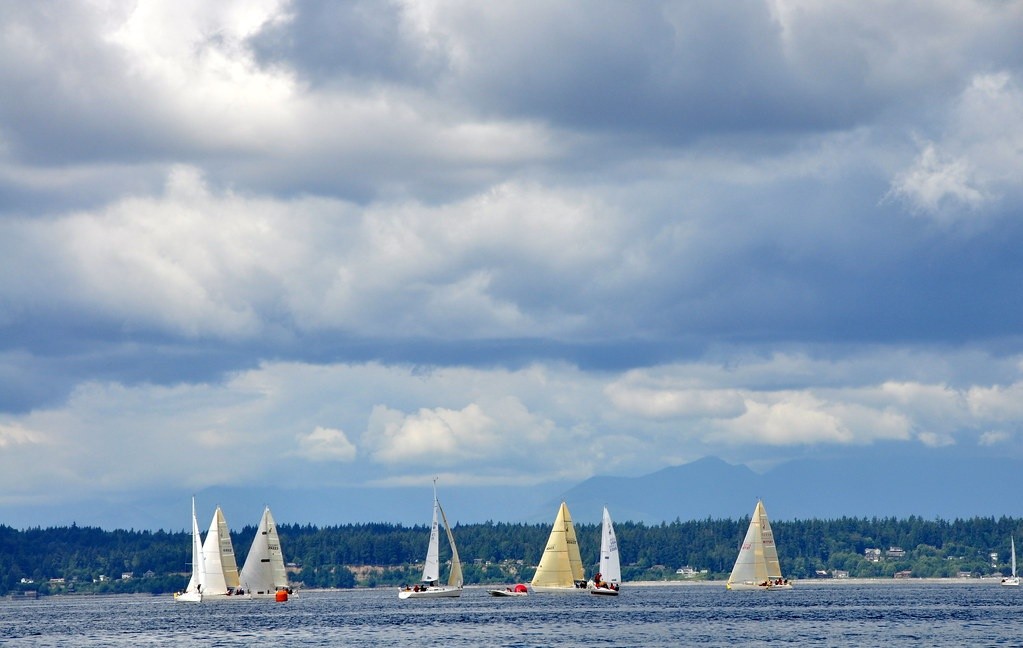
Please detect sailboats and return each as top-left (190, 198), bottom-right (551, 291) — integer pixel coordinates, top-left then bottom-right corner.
top-left (530, 499), bottom-right (591, 593)
top-left (173, 494), bottom-right (299, 603)
top-left (1001, 536), bottom-right (1021, 586)
top-left (398, 476), bottom-right (466, 599)
top-left (726, 500), bottom-right (795, 591)
top-left (591, 505), bottom-right (623, 595)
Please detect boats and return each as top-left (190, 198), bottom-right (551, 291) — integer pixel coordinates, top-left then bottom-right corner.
top-left (484, 584), bottom-right (529, 598)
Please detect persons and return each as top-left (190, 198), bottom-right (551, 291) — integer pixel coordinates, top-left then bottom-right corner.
top-left (178, 588), bottom-right (186, 595)
top-left (414, 585), bottom-right (424, 592)
top-left (1002, 579), bottom-right (1005, 582)
top-left (595, 580), bottom-right (619, 591)
top-left (784, 579), bottom-right (787, 585)
top-left (235, 588), bottom-right (244, 595)
top-left (226, 588), bottom-right (233, 596)
top-left (406, 584), bottom-right (410, 590)
top-left (507, 588), bottom-right (512, 592)
top-left (762, 581), bottom-right (767, 586)
top-left (775, 578), bottom-right (782, 585)
top-left (282, 587), bottom-right (293, 594)
top-left (576, 581), bottom-right (587, 589)
